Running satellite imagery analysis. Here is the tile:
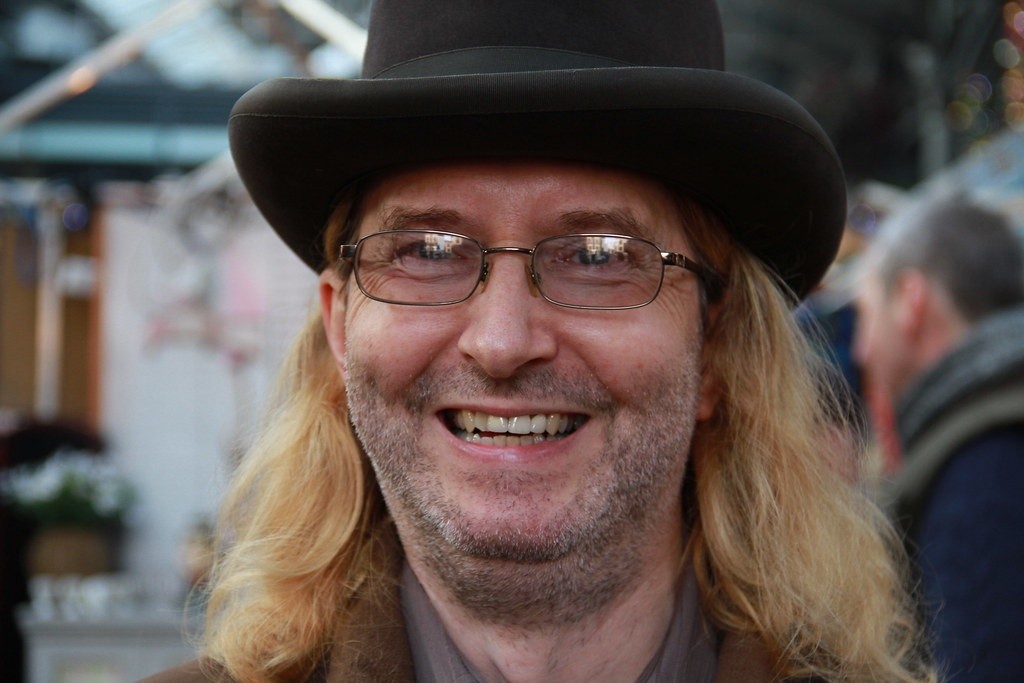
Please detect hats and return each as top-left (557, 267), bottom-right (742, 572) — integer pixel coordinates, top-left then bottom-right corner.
top-left (227, 0), bottom-right (846, 312)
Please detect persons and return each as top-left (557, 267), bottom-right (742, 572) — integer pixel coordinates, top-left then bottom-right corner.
top-left (852, 187), bottom-right (1024, 683)
top-left (137, 2), bottom-right (930, 683)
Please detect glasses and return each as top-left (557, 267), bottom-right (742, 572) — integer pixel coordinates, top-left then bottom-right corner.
top-left (339, 229), bottom-right (711, 310)
